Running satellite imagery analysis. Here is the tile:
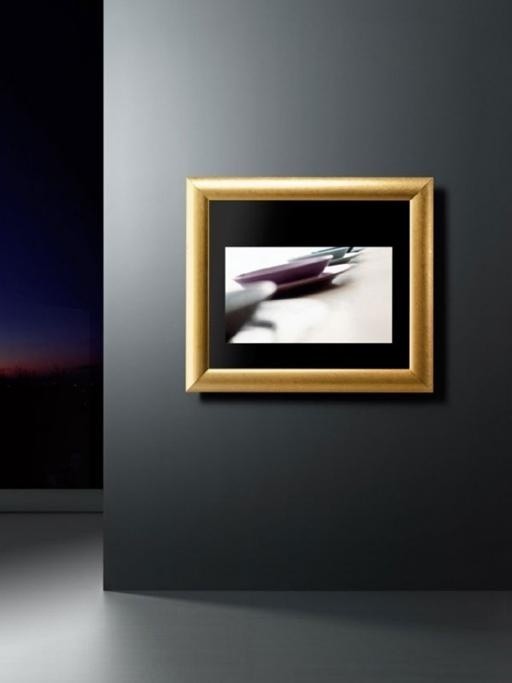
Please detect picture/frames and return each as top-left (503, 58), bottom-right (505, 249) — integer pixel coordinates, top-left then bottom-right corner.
top-left (185, 176), bottom-right (433, 394)
